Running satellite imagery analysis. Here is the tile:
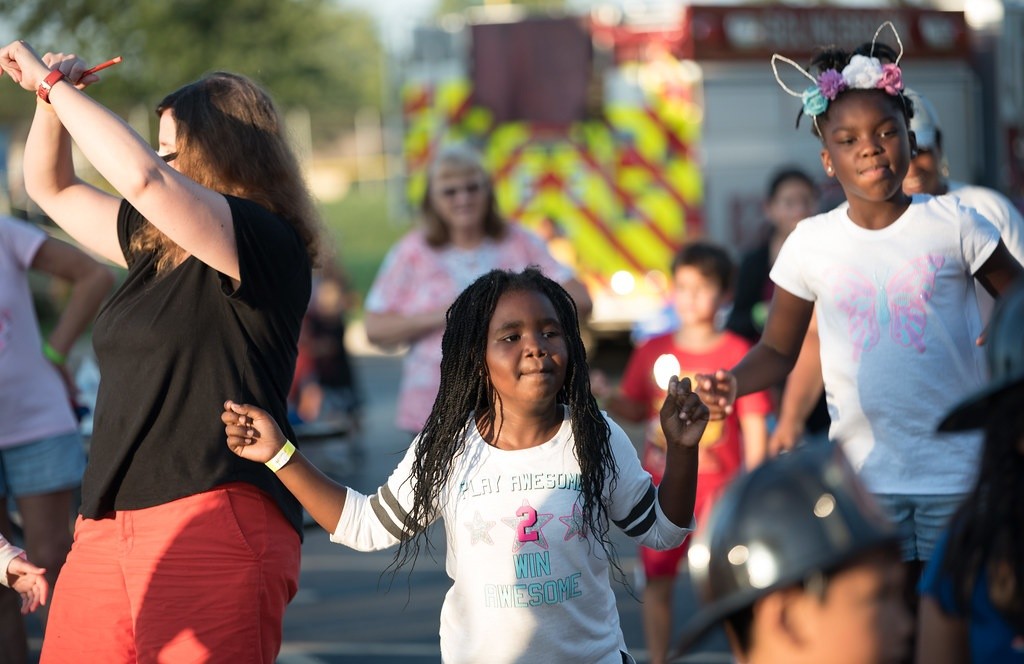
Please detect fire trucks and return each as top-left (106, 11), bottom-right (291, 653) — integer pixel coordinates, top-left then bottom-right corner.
top-left (400, 0), bottom-right (1023, 364)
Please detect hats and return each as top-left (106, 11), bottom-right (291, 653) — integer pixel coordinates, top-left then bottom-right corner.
top-left (904, 86), bottom-right (944, 148)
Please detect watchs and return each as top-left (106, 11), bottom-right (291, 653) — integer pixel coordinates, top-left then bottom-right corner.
top-left (38, 69), bottom-right (73, 104)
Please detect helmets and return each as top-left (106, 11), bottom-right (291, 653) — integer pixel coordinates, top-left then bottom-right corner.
top-left (666, 437), bottom-right (897, 662)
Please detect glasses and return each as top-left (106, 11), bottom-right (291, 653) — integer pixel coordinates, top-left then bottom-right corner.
top-left (440, 184), bottom-right (479, 198)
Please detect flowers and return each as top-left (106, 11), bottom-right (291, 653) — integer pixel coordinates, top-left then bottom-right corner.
top-left (801, 55), bottom-right (904, 116)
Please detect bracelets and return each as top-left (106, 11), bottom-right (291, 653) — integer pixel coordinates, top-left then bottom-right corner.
top-left (44, 344), bottom-right (64, 366)
top-left (265, 440), bottom-right (296, 472)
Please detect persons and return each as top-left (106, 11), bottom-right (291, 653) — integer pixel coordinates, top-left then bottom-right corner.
top-left (0, 209), bottom-right (115, 664)
top-left (666, 448), bottom-right (912, 664)
top-left (590, 240), bottom-right (773, 664)
top-left (692, 41), bottom-right (1023, 663)
top-left (288, 238), bottom-right (359, 436)
top-left (222, 270), bottom-right (710, 663)
top-left (722, 170), bottom-right (830, 440)
top-left (363, 144), bottom-right (593, 432)
top-left (0, 40), bottom-right (325, 664)
top-left (0, 533), bottom-right (49, 614)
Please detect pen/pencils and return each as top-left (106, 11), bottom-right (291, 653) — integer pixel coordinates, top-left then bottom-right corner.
top-left (81, 56), bottom-right (122, 78)
